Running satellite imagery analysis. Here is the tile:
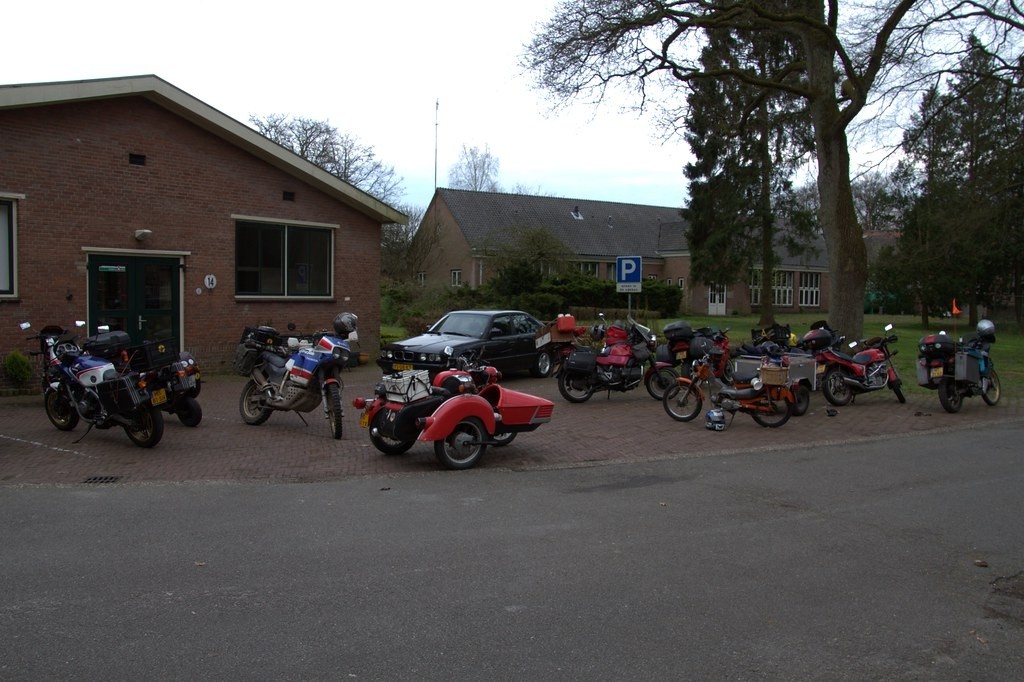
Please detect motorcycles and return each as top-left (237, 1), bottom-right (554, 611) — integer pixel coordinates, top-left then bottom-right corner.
top-left (533, 311), bottom-right (681, 404)
top-left (662, 321), bottom-right (736, 384)
top-left (802, 322), bottom-right (907, 408)
top-left (350, 348), bottom-right (555, 474)
top-left (232, 312), bottom-right (360, 440)
top-left (19, 320), bottom-right (202, 448)
top-left (729, 313), bottom-right (845, 418)
top-left (914, 320), bottom-right (1002, 414)
top-left (662, 343), bottom-right (794, 429)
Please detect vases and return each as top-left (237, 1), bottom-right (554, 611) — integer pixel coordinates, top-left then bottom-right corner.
top-left (358, 353), bottom-right (369, 366)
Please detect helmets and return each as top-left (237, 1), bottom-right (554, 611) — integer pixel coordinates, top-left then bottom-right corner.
top-left (705, 409), bottom-right (726, 431)
top-left (789, 333), bottom-right (797, 346)
top-left (590, 324), bottom-right (604, 341)
top-left (334, 312), bottom-right (359, 334)
top-left (976, 319), bottom-right (995, 336)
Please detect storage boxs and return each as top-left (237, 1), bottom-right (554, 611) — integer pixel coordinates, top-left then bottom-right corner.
top-left (232, 344), bottom-right (256, 377)
top-left (381, 369), bottom-right (433, 403)
top-left (657, 345), bottom-right (681, 367)
top-left (88, 329), bottom-right (130, 357)
top-left (760, 367), bottom-right (788, 385)
top-left (751, 324), bottom-right (790, 345)
top-left (240, 326), bottom-right (283, 350)
top-left (955, 353), bottom-right (979, 383)
top-left (96, 371), bottom-right (149, 413)
top-left (689, 337), bottom-right (713, 358)
top-left (916, 358), bottom-right (936, 390)
top-left (125, 337), bottom-right (181, 371)
top-left (566, 352), bottom-right (596, 373)
top-left (534, 319), bottom-right (573, 349)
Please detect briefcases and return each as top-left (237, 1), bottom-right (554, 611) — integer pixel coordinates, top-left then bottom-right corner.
top-left (761, 367), bottom-right (788, 385)
top-left (382, 369), bottom-right (433, 403)
top-left (751, 324), bottom-right (790, 346)
top-left (126, 337), bottom-right (177, 371)
top-left (241, 326), bottom-right (283, 346)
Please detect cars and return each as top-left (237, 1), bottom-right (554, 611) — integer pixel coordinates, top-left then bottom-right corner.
top-left (376, 309), bottom-right (562, 378)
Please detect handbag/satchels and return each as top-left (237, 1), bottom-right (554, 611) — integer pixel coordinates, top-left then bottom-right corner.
top-left (606, 327), bottom-right (628, 345)
top-left (632, 341), bottom-right (649, 359)
